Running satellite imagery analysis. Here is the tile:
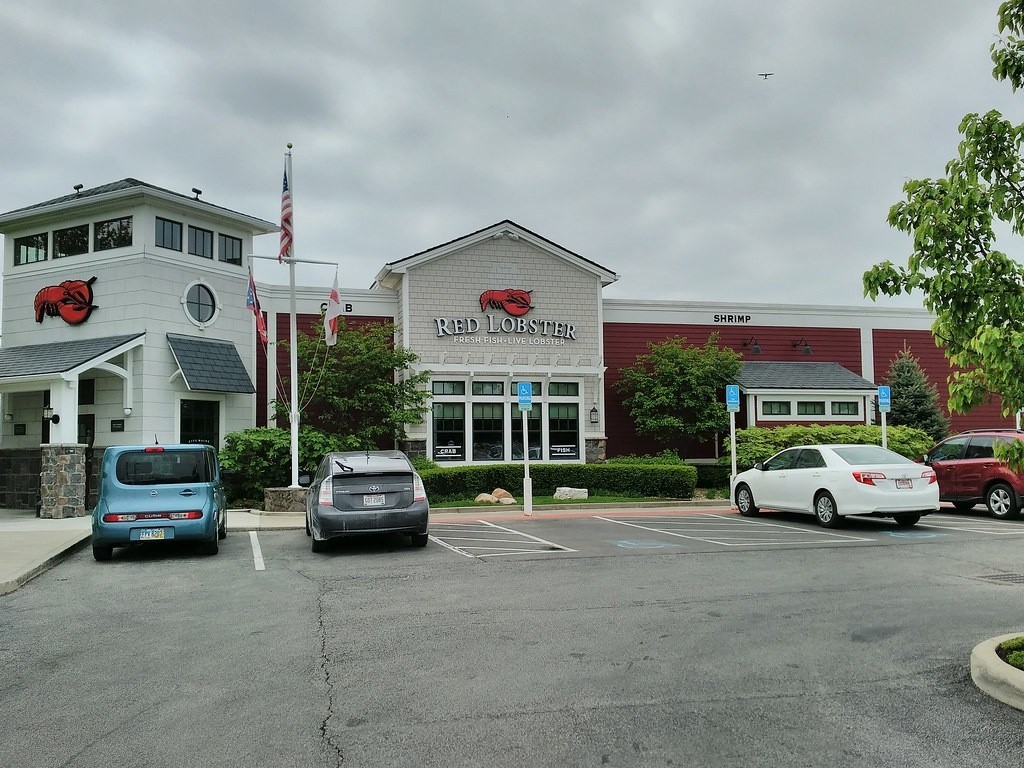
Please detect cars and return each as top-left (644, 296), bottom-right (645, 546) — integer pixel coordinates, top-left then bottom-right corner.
top-left (306, 449), bottom-right (429, 554)
top-left (731, 443), bottom-right (940, 528)
top-left (92, 444), bottom-right (228, 563)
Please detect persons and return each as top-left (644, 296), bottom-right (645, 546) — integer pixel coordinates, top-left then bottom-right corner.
top-left (323, 274), bottom-right (342, 346)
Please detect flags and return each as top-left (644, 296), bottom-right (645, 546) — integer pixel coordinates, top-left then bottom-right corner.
top-left (277, 156), bottom-right (292, 263)
top-left (245, 266), bottom-right (268, 344)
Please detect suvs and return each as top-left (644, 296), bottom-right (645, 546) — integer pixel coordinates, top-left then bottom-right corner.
top-left (913, 428), bottom-right (1024, 519)
